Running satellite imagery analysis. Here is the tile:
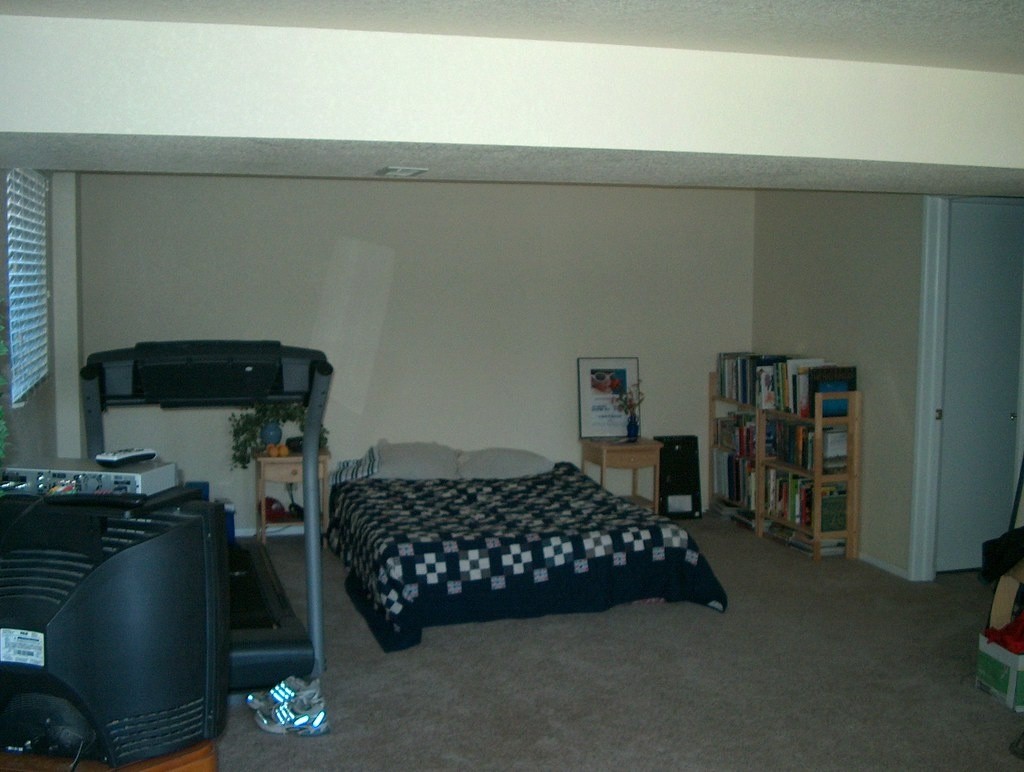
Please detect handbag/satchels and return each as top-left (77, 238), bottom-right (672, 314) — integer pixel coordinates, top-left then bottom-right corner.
top-left (981, 526), bottom-right (1024, 584)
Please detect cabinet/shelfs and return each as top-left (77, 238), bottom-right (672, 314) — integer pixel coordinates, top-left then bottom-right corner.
top-left (709, 371), bottom-right (862, 561)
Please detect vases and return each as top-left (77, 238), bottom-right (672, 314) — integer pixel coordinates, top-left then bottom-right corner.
top-left (628, 415), bottom-right (639, 437)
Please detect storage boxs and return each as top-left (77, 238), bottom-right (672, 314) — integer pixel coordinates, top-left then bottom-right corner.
top-left (974, 632), bottom-right (1024, 712)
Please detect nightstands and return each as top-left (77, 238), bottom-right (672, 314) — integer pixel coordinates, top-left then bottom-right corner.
top-left (253, 446), bottom-right (331, 543)
top-left (581, 438), bottom-right (664, 514)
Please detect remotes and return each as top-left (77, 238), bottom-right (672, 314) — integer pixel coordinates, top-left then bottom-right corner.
top-left (95, 448), bottom-right (157, 469)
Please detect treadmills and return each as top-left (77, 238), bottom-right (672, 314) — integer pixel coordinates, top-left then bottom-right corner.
top-left (72, 335), bottom-right (337, 713)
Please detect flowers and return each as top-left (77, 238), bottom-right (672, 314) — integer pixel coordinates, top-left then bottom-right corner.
top-left (612, 379), bottom-right (646, 416)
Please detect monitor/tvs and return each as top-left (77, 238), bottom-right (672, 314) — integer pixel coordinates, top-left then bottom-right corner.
top-left (0, 485), bottom-right (227, 768)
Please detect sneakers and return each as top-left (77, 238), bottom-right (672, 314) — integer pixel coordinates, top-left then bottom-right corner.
top-left (253, 691), bottom-right (329, 736)
top-left (246, 676), bottom-right (328, 711)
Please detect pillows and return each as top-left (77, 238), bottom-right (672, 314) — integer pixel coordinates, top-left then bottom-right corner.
top-left (330, 447), bottom-right (380, 485)
top-left (458, 447), bottom-right (556, 480)
top-left (357, 440), bottom-right (462, 482)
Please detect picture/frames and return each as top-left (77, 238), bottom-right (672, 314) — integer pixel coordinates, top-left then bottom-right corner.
top-left (577, 357), bottom-right (640, 440)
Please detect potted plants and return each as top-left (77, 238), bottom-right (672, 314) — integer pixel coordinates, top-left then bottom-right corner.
top-left (229, 402), bottom-right (330, 468)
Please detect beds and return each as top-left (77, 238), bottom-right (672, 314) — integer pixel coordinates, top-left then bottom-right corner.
top-left (326, 462), bottom-right (727, 653)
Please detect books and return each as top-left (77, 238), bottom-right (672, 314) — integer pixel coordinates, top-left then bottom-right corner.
top-left (709, 448), bottom-right (847, 556)
top-left (714, 411), bottom-right (775, 457)
top-left (775, 420), bottom-right (849, 473)
top-left (717, 352), bottom-right (857, 418)
